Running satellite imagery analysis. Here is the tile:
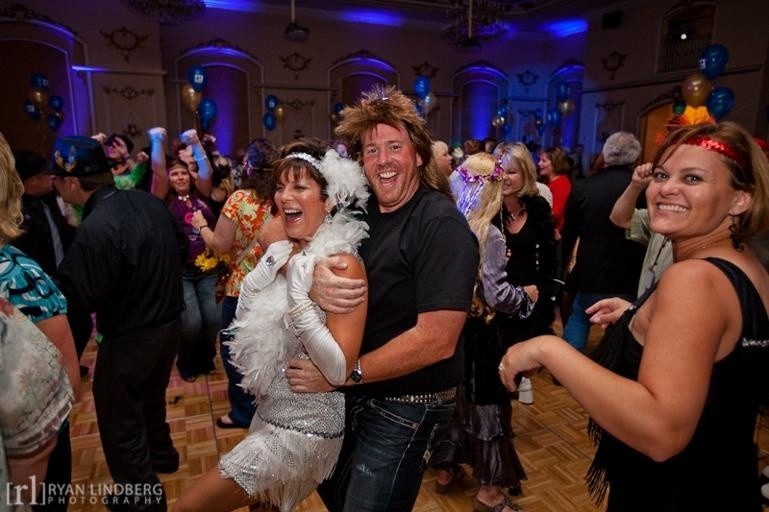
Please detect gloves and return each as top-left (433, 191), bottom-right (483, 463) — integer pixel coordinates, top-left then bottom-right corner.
top-left (286, 250), bottom-right (346, 386)
top-left (235, 240), bottom-right (293, 320)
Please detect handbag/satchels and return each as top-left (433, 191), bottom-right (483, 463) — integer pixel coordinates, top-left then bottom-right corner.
top-left (215, 267), bottom-right (232, 304)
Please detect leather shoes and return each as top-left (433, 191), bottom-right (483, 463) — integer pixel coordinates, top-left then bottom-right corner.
top-left (518, 376), bottom-right (534, 405)
top-left (105, 491), bottom-right (166, 512)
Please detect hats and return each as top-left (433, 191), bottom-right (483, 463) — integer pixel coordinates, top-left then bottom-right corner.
top-left (41, 135), bottom-right (118, 177)
top-left (13, 150), bottom-right (47, 180)
top-left (105, 133), bottom-right (133, 153)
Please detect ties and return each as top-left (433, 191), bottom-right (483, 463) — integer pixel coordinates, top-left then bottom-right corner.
top-left (38, 198), bottom-right (64, 270)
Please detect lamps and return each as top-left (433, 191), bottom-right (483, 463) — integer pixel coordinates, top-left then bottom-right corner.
top-left (440, 0), bottom-right (508, 51)
top-left (285, 0), bottom-right (309, 41)
top-left (128, 0), bottom-right (206, 25)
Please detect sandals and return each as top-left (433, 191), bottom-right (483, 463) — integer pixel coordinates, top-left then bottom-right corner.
top-left (471, 494), bottom-right (524, 511)
top-left (177, 363), bottom-right (217, 381)
top-left (434, 464), bottom-right (465, 494)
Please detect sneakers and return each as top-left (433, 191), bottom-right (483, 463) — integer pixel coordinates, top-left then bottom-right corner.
top-left (217, 411), bottom-right (250, 428)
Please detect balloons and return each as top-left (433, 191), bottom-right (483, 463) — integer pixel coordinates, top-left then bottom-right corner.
top-left (681, 71), bottom-right (711, 108)
top-left (188, 62), bottom-right (208, 93)
top-left (412, 77), bottom-right (436, 119)
top-left (492, 97), bottom-right (576, 139)
top-left (22, 70), bottom-right (66, 130)
top-left (697, 43), bottom-right (728, 81)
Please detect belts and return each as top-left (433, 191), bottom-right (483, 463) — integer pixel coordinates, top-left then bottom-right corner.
top-left (384, 386), bottom-right (457, 405)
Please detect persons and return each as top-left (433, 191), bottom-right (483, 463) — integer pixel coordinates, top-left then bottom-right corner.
top-left (0, 83), bottom-right (765, 512)
